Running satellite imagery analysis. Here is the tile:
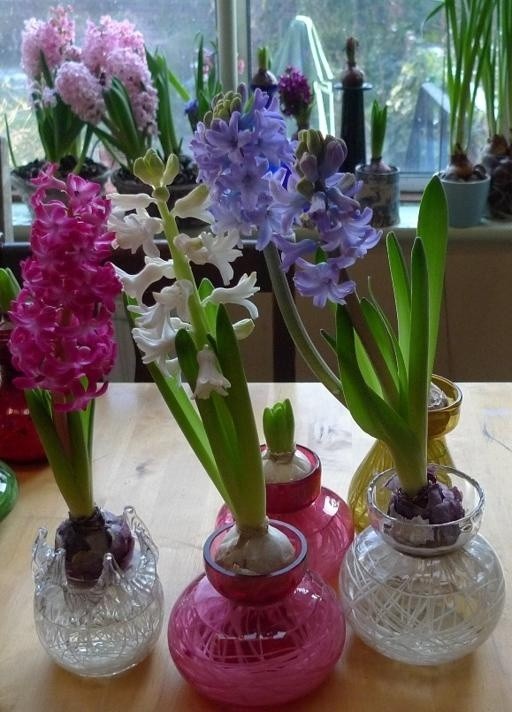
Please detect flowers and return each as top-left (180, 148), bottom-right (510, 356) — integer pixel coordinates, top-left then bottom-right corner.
top-left (13, 3), bottom-right (103, 175)
top-left (276, 67), bottom-right (321, 136)
top-left (185, 87), bottom-right (474, 545)
top-left (3, 160), bottom-right (134, 590)
top-left (51, 16), bottom-right (155, 181)
top-left (98, 135), bottom-right (288, 575)
top-left (182, 38), bottom-right (244, 164)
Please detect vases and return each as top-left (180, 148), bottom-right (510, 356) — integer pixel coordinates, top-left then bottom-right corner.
top-left (344, 463), bottom-right (503, 667)
top-left (27, 511), bottom-right (162, 680)
top-left (11, 154), bottom-right (109, 222)
top-left (169, 521), bottom-right (345, 705)
top-left (109, 157), bottom-right (205, 232)
top-left (204, 400), bottom-right (352, 602)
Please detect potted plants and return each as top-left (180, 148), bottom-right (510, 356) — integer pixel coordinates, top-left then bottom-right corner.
top-left (356, 97), bottom-right (401, 229)
top-left (429, 1), bottom-right (490, 228)
top-left (322, 368), bottom-right (463, 550)
top-left (467, 1), bottom-right (511, 223)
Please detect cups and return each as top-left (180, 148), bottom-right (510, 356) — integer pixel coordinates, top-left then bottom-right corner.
top-left (355, 164), bottom-right (403, 228)
top-left (433, 172), bottom-right (494, 228)
